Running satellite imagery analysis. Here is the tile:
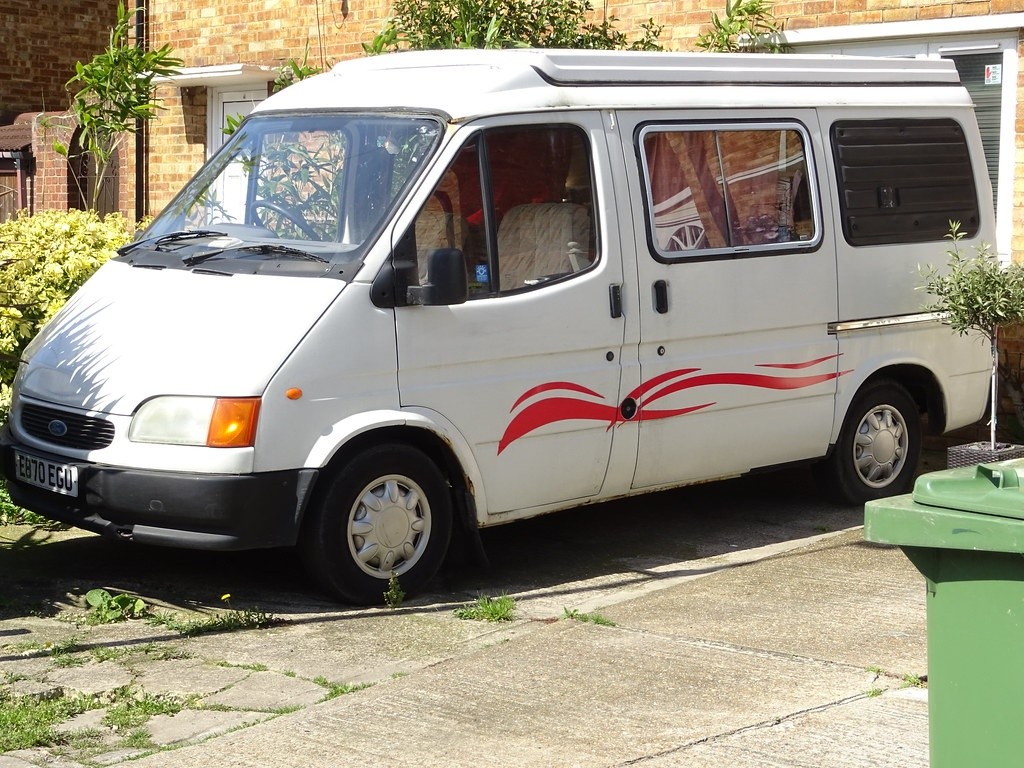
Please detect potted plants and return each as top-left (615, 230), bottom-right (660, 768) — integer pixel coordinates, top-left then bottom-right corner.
top-left (922, 220), bottom-right (1024, 468)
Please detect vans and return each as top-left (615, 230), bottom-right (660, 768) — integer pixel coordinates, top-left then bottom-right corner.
top-left (1, 47), bottom-right (1000, 609)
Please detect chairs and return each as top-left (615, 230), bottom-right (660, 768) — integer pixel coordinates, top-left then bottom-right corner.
top-left (497, 203), bottom-right (578, 296)
top-left (414, 169), bottom-right (462, 279)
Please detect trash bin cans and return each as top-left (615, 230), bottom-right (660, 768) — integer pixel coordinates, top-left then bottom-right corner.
top-left (863, 460), bottom-right (1024, 768)
